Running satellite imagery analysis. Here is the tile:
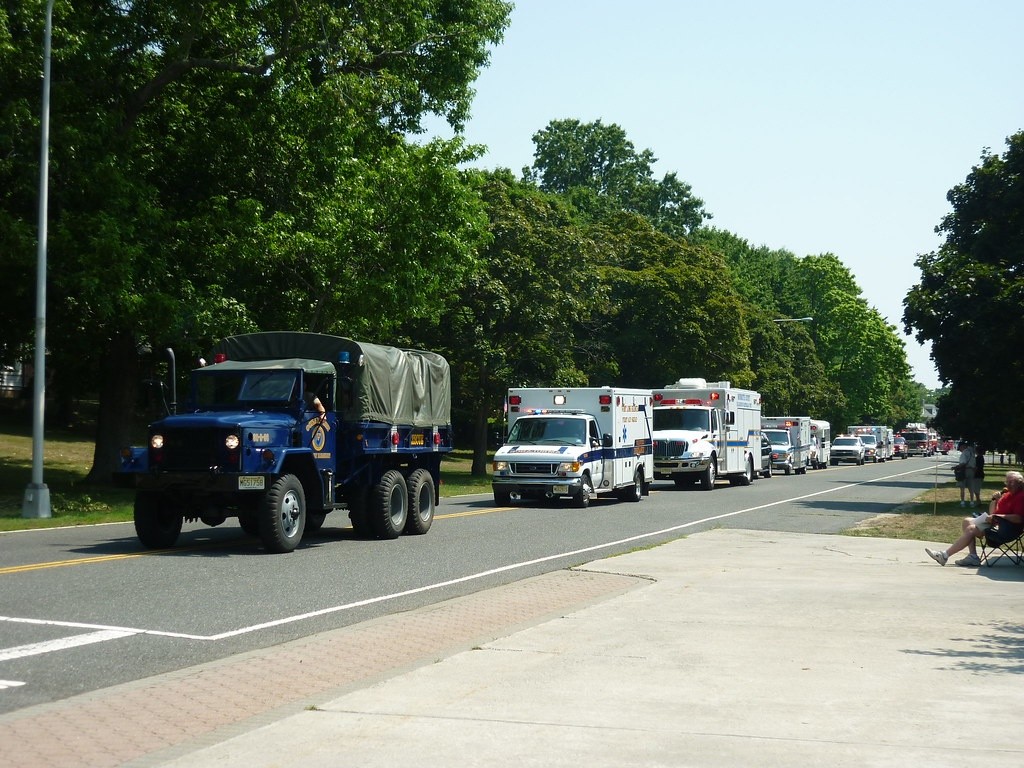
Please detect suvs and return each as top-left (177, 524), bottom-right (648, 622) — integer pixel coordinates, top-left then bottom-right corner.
top-left (761, 432), bottom-right (774, 479)
top-left (895, 434), bottom-right (909, 460)
top-left (830, 436), bottom-right (866, 466)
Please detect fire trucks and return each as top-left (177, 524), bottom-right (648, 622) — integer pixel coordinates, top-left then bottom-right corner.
top-left (929, 427), bottom-right (954, 455)
top-left (896, 423), bottom-right (934, 457)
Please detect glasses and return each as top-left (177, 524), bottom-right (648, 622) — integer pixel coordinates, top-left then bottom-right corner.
top-left (1004, 481), bottom-right (1014, 485)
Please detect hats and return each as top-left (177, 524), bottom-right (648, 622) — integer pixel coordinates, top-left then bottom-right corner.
top-left (957, 442), bottom-right (965, 451)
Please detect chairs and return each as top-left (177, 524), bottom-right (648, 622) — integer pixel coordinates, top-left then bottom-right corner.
top-left (972, 512), bottom-right (1024, 567)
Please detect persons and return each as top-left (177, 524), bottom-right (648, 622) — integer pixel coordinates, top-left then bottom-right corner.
top-left (293, 381), bottom-right (326, 423)
top-left (955, 442), bottom-right (985, 508)
top-left (925, 470), bottom-right (1024, 567)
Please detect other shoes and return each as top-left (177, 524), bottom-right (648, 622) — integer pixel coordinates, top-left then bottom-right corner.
top-left (970, 503), bottom-right (975, 508)
top-left (960, 503), bottom-right (966, 507)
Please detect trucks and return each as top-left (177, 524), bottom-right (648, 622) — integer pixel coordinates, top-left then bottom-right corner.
top-left (130, 332), bottom-right (454, 554)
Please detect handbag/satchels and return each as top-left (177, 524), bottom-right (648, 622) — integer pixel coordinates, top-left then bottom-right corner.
top-left (974, 470), bottom-right (985, 479)
top-left (954, 465), bottom-right (966, 482)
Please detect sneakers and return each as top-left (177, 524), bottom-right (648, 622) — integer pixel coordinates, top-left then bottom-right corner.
top-left (955, 554), bottom-right (980, 566)
top-left (925, 547), bottom-right (947, 566)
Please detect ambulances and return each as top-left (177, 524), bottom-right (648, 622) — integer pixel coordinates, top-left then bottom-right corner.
top-left (887, 428), bottom-right (896, 460)
top-left (809, 418), bottom-right (832, 470)
top-left (648, 378), bottom-right (762, 490)
top-left (762, 415), bottom-right (812, 475)
top-left (847, 425), bottom-right (888, 464)
top-left (492, 386), bottom-right (654, 510)
